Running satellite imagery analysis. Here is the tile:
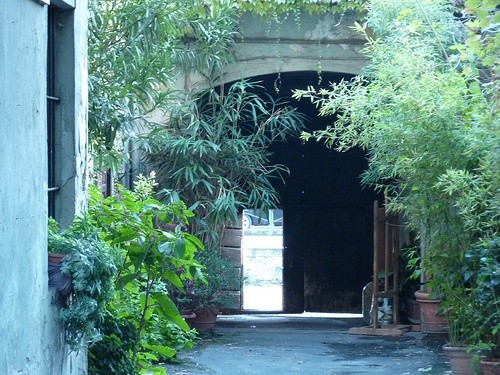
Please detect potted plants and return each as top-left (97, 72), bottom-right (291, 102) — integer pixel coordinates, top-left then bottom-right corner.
top-left (195, 277), bottom-right (227, 331)
top-left (406, 246), bottom-right (463, 334)
top-left (171, 277), bottom-right (196, 328)
top-left (48, 216), bottom-right (98, 268)
top-left (464, 324), bottom-right (499, 375)
top-left (429, 289), bottom-right (492, 374)
